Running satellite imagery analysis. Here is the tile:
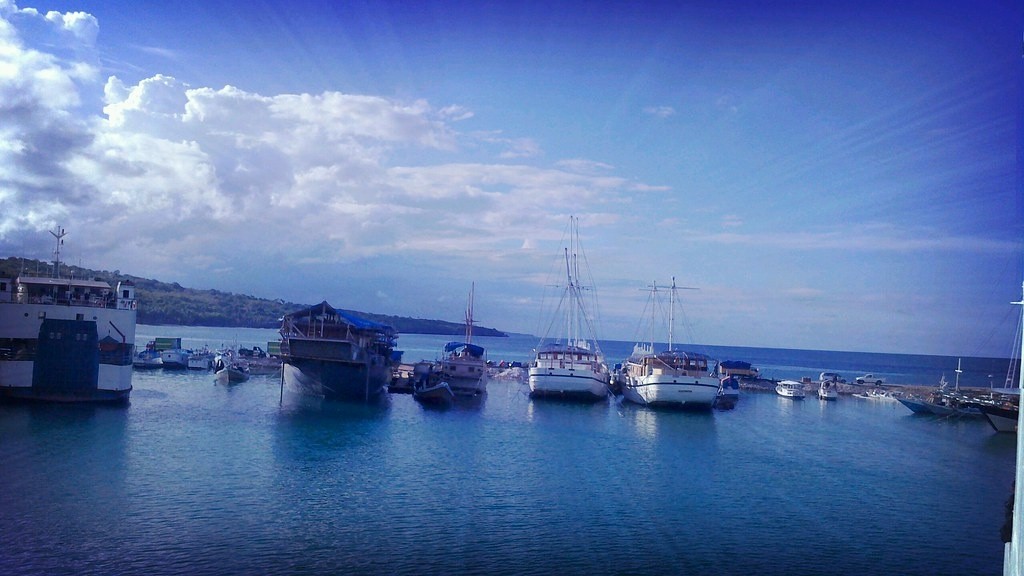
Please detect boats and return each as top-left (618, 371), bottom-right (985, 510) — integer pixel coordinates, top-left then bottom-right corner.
top-left (894, 265), bottom-right (1024, 435)
top-left (774, 381), bottom-right (805, 399)
top-left (817, 380), bottom-right (838, 400)
top-left (122, 212), bottom-right (740, 416)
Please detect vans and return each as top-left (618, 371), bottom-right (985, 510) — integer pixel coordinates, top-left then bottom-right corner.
top-left (819, 373), bottom-right (848, 384)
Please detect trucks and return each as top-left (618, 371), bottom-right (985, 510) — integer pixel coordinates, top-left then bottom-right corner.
top-left (721, 360), bottom-right (758, 378)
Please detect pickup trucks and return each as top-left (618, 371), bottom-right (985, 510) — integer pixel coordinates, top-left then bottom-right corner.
top-left (856, 373), bottom-right (887, 385)
